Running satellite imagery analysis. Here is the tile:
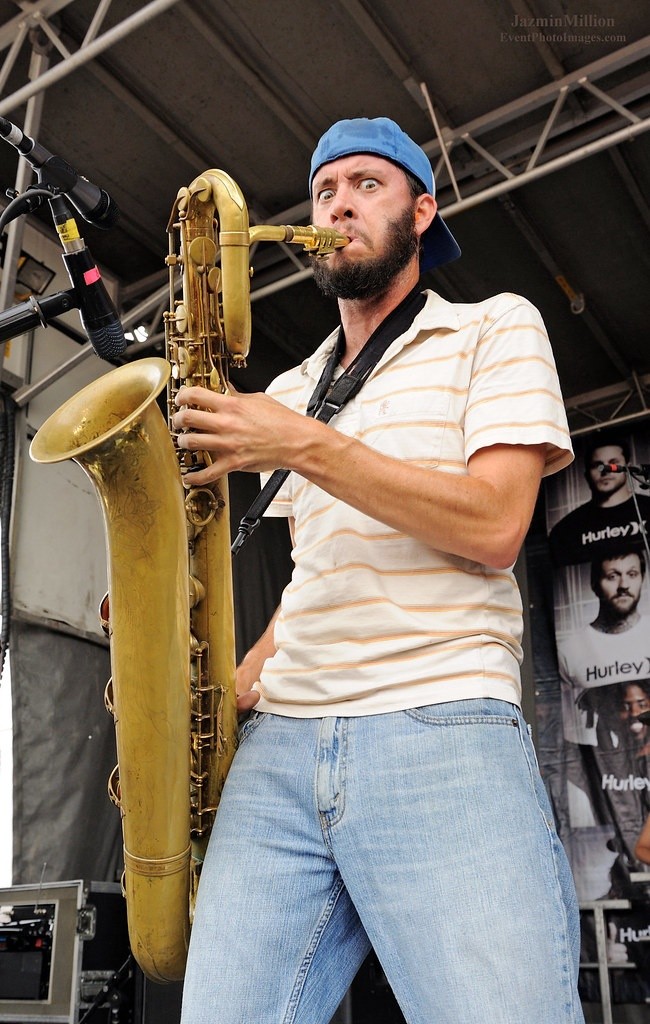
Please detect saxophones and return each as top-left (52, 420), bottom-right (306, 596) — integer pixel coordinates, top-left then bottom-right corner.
top-left (31, 164), bottom-right (351, 987)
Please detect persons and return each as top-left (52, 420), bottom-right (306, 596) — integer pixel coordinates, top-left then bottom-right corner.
top-left (173, 117), bottom-right (587, 1024)
top-left (634, 813), bottom-right (650, 866)
top-left (550, 437), bottom-right (650, 830)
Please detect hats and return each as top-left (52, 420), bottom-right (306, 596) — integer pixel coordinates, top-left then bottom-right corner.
top-left (309, 117), bottom-right (461, 268)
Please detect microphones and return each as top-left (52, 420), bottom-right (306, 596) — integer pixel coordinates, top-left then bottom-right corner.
top-left (46, 195), bottom-right (127, 360)
top-left (0, 117), bottom-right (122, 231)
top-left (634, 464), bottom-right (650, 476)
